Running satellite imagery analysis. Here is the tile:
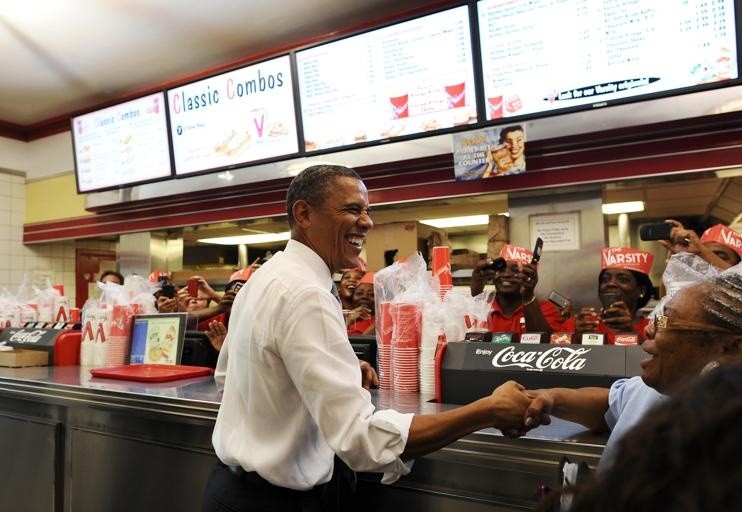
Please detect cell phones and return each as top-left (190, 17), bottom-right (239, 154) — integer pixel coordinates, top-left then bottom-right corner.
top-left (531, 237), bottom-right (543, 264)
top-left (481, 258), bottom-right (507, 279)
top-left (548, 291), bottom-right (571, 308)
top-left (606, 295), bottom-right (624, 327)
top-left (639, 222), bottom-right (678, 241)
top-left (162, 286), bottom-right (175, 299)
top-left (187, 280), bottom-right (198, 297)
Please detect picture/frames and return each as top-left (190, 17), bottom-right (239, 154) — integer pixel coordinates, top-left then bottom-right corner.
top-left (528, 208), bottom-right (585, 255)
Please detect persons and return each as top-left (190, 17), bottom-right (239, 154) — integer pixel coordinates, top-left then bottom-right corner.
top-left (496, 270), bottom-right (740, 441)
top-left (480, 123), bottom-right (529, 178)
top-left (654, 217), bottom-right (740, 300)
top-left (516, 354), bottom-right (741, 510)
top-left (197, 162), bottom-right (552, 512)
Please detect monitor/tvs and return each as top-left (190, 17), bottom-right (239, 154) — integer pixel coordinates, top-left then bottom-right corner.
top-left (346, 335), bottom-right (378, 389)
top-left (291, 4), bottom-right (483, 157)
top-left (164, 50), bottom-right (301, 179)
top-left (69, 86), bottom-right (175, 194)
top-left (472, 0), bottom-right (742, 128)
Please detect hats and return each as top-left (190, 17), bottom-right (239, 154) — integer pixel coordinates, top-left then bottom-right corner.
top-left (500, 244), bottom-right (540, 264)
top-left (699, 223), bottom-right (742, 260)
top-left (600, 247), bottom-right (654, 277)
top-left (228, 268), bottom-right (256, 286)
top-left (355, 272), bottom-right (377, 289)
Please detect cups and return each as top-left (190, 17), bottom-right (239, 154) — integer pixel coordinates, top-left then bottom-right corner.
top-left (390, 94), bottom-right (409, 120)
top-left (491, 143), bottom-right (515, 169)
top-left (444, 81), bottom-right (466, 109)
top-left (342, 308), bottom-right (357, 332)
top-left (487, 94), bottom-right (503, 120)
top-left (376, 305), bottom-right (458, 395)
top-left (1, 301), bottom-right (81, 329)
top-left (81, 291), bottom-right (153, 368)
top-left (430, 246), bottom-right (454, 300)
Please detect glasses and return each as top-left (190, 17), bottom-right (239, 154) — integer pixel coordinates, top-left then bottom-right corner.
top-left (653, 312), bottom-right (733, 336)
